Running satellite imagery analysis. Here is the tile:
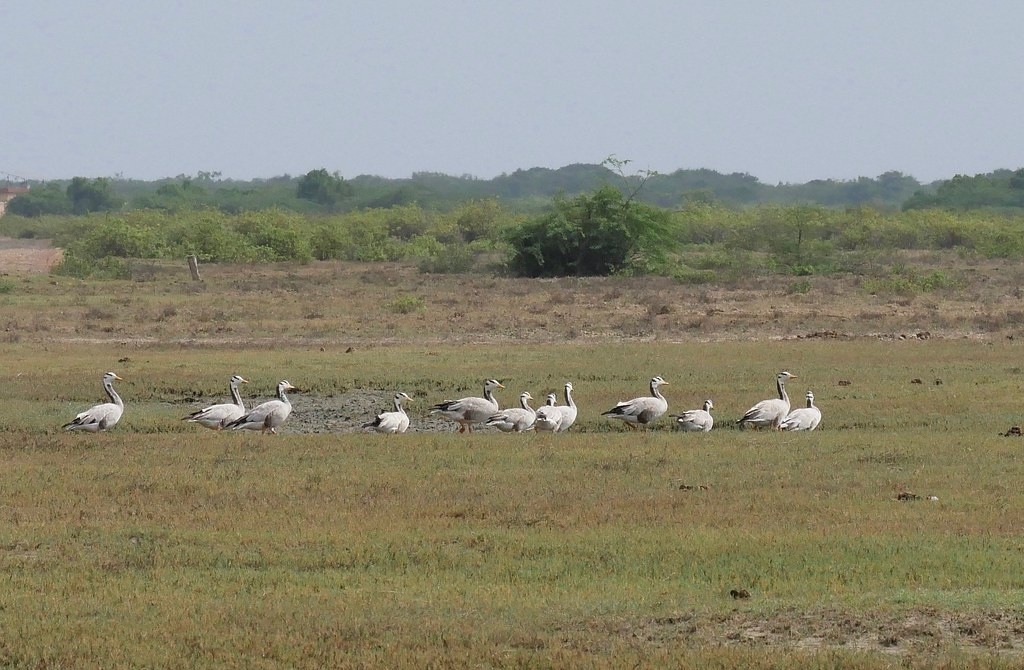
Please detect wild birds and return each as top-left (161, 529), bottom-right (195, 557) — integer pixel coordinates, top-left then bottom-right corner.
top-left (61, 372), bottom-right (124, 433)
top-left (601, 374), bottom-right (670, 433)
top-left (428, 379), bottom-right (578, 436)
top-left (735, 370), bottom-right (798, 431)
top-left (778, 390), bottom-right (821, 431)
top-left (361, 392), bottom-right (414, 438)
top-left (224, 379), bottom-right (295, 435)
top-left (181, 375), bottom-right (249, 433)
top-left (668, 398), bottom-right (715, 432)
